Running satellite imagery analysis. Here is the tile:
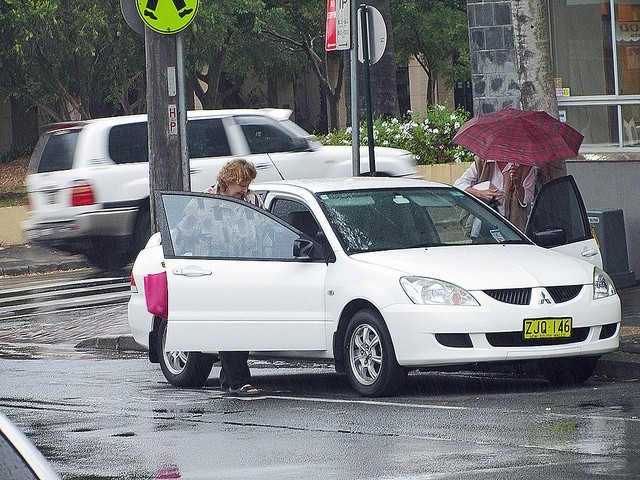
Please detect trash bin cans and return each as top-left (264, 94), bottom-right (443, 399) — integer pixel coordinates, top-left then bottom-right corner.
top-left (586, 208), bottom-right (634, 290)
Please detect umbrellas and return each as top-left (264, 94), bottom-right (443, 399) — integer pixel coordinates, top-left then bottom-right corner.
top-left (450, 111), bottom-right (584, 188)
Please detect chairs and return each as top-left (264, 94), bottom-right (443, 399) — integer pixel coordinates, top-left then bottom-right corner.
top-left (285, 209), bottom-right (335, 261)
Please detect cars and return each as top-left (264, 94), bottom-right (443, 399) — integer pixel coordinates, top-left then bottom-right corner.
top-left (130, 176), bottom-right (624, 395)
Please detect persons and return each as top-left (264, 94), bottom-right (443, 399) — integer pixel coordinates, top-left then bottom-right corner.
top-left (169, 160), bottom-right (272, 397)
top-left (451, 155), bottom-right (538, 247)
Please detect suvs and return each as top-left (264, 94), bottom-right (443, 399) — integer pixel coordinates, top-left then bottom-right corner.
top-left (20, 108), bottom-right (418, 270)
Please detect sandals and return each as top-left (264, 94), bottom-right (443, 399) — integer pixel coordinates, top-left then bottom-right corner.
top-left (228, 384), bottom-right (260, 395)
